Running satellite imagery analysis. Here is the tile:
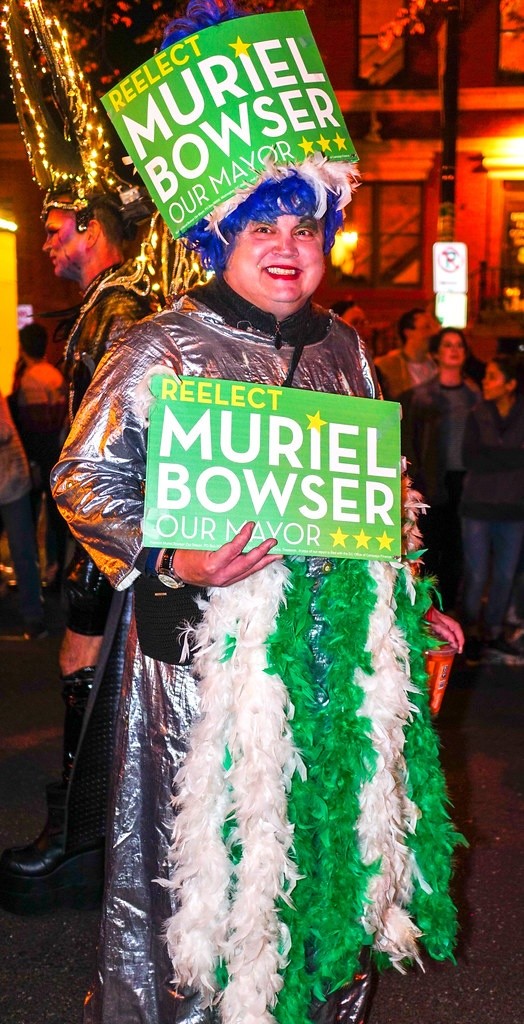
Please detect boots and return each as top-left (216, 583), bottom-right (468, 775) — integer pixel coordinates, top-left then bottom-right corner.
top-left (1, 781), bottom-right (105, 914)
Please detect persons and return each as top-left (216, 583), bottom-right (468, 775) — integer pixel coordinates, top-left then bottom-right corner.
top-left (1, 0), bottom-right (524, 1024)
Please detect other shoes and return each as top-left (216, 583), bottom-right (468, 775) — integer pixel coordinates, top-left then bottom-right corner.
top-left (483, 635), bottom-right (523, 658)
top-left (28, 619), bottom-right (49, 639)
top-left (464, 632), bottom-right (482, 665)
top-left (6, 580), bottom-right (19, 593)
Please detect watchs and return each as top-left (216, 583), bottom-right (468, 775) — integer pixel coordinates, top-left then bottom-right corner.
top-left (157, 547), bottom-right (187, 592)
